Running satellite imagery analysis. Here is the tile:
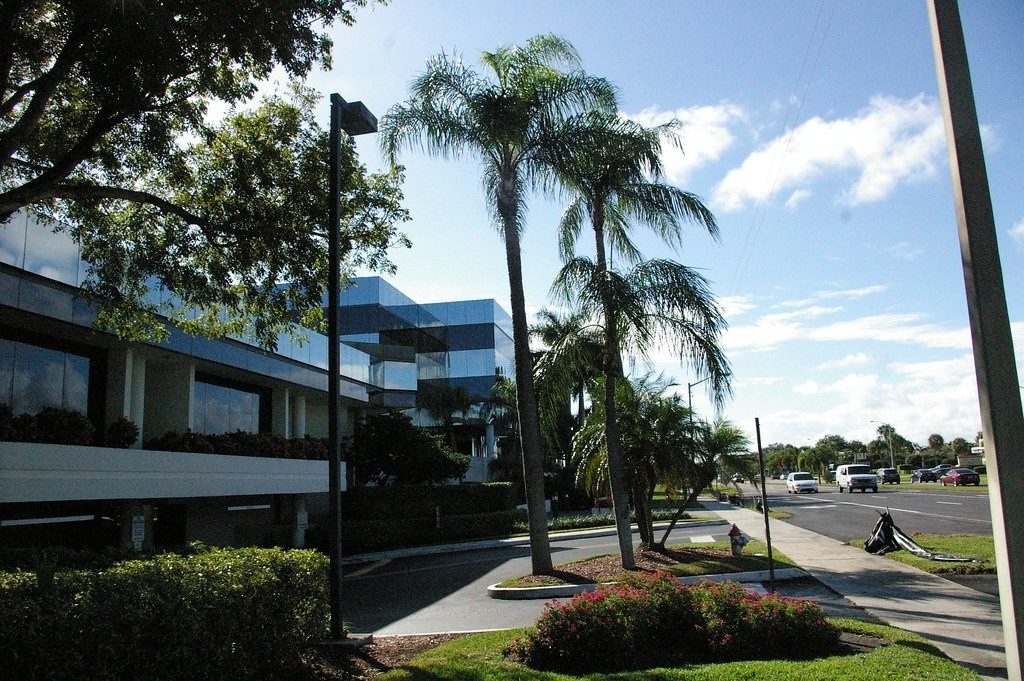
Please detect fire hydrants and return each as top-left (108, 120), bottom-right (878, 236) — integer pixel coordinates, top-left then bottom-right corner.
top-left (727, 523), bottom-right (749, 557)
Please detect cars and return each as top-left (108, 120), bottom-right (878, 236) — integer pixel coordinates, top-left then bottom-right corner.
top-left (836, 464), bottom-right (879, 493)
top-left (715, 472), bottom-right (746, 484)
top-left (935, 463), bottom-right (954, 472)
top-left (939, 467), bottom-right (981, 488)
top-left (910, 468), bottom-right (937, 483)
top-left (787, 471), bottom-right (819, 493)
top-left (876, 466), bottom-right (901, 484)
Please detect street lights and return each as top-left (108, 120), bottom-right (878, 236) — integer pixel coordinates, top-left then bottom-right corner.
top-left (688, 372), bottom-right (731, 498)
top-left (870, 421), bottom-right (894, 468)
top-left (314, 93), bottom-right (382, 647)
